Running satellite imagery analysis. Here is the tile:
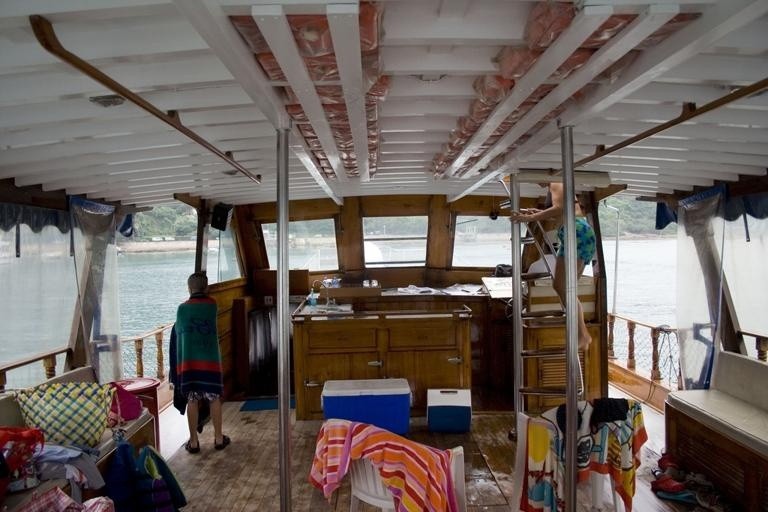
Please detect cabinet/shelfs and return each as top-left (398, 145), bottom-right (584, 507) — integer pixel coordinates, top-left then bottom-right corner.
top-left (291, 295), bottom-right (603, 420)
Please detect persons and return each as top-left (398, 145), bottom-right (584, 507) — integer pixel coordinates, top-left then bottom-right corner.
top-left (507, 181), bottom-right (599, 354)
top-left (168, 271), bottom-right (231, 455)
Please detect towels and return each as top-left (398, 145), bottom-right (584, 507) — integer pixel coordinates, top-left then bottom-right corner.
top-left (167, 292), bottom-right (225, 432)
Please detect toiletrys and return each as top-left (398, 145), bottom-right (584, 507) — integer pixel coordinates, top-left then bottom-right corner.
top-left (308, 288), bottom-right (317, 306)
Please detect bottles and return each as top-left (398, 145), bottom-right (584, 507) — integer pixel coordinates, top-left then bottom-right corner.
top-left (322, 276), bottom-right (339, 288)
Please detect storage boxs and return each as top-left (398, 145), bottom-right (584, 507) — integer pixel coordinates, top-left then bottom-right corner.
top-left (318, 378), bottom-right (472, 435)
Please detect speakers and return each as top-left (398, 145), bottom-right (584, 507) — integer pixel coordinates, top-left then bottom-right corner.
top-left (210, 202), bottom-right (234, 232)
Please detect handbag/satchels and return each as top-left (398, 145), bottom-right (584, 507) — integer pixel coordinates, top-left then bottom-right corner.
top-left (0, 381), bottom-right (124, 448)
top-left (16, 485), bottom-right (116, 511)
top-left (97, 442), bottom-right (188, 511)
top-left (110, 382), bottom-right (155, 420)
top-left (0, 425), bottom-right (46, 493)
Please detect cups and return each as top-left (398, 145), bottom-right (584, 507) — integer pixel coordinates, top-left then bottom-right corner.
top-left (363, 280), bottom-right (378, 288)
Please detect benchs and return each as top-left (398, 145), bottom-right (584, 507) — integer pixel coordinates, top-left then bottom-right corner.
top-left (665, 352), bottom-right (768, 511)
top-left (0, 367), bottom-right (157, 510)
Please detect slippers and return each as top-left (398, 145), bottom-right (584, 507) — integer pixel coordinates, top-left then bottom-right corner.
top-left (185, 439), bottom-right (201, 452)
top-left (214, 434), bottom-right (230, 450)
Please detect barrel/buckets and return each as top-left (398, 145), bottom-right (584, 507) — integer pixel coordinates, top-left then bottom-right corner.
top-left (110, 377), bottom-right (160, 451)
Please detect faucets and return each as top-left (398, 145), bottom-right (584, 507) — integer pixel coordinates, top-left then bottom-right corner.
top-left (312, 279), bottom-right (330, 306)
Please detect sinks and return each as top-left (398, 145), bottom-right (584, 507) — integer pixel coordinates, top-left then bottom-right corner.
top-left (299, 303), bottom-right (355, 317)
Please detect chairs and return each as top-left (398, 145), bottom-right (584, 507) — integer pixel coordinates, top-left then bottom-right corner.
top-left (510, 399), bottom-right (645, 512)
top-left (309, 419), bottom-right (468, 512)
